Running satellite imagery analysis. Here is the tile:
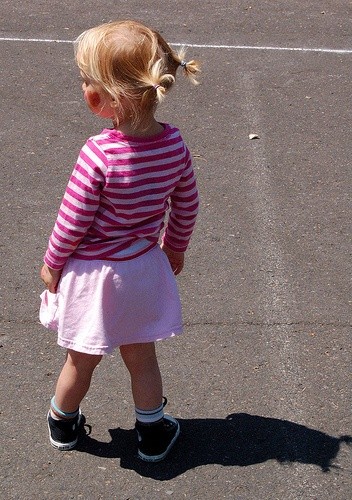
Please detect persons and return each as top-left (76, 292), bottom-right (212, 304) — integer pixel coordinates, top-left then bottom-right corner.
top-left (38, 21), bottom-right (201, 462)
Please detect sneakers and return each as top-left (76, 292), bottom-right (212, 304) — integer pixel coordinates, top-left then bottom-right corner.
top-left (136, 411), bottom-right (180, 460)
top-left (47, 406), bottom-right (83, 451)
